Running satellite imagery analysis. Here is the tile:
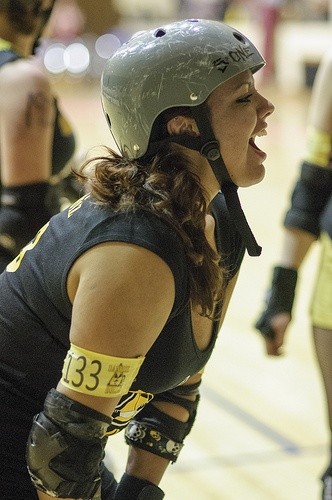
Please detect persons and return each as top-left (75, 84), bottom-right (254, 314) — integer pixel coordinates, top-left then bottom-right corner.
top-left (252, 34), bottom-right (331, 500)
top-left (0, 0), bottom-right (78, 272)
top-left (0, 17), bottom-right (275, 499)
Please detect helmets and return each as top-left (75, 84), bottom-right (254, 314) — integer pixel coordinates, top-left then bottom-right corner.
top-left (101, 20), bottom-right (267, 160)
